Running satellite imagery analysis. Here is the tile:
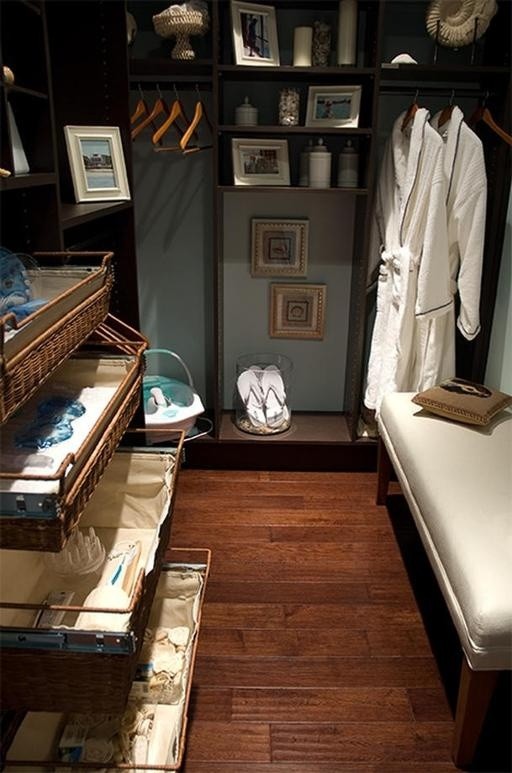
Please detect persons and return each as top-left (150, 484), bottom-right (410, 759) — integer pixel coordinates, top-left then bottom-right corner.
top-left (247, 17), bottom-right (265, 57)
top-left (321, 98), bottom-right (333, 118)
top-left (244, 155), bottom-right (278, 174)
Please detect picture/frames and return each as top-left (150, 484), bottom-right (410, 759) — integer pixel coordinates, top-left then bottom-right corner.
top-left (230, 1), bottom-right (282, 68)
top-left (63, 125), bottom-right (132, 203)
top-left (231, 138), bottom-right (291, 187)
top-left (250, 218), bottom-right (327, 340)
top-left (304, 86), bottom-right (362, 129)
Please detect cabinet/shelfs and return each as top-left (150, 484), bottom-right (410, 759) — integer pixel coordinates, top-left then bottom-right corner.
top-left (1, 2), bottom-right (140, 353)
top-left (127, 0), bottom-right (512, 471)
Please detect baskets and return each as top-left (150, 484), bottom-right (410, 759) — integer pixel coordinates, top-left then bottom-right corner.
top-left (151, 11), bottom-right (209, 60)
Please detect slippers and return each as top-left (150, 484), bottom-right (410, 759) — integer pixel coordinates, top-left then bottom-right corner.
top-left (261, 370), bottom-right (285, 427)
top-left (237, 370), bottom-right (266, 426)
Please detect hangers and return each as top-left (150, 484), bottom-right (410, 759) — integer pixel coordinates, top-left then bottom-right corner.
top-left (130, 82), bottom-right (215, 157)
top-left (401, 89), bottom-right (512, 153)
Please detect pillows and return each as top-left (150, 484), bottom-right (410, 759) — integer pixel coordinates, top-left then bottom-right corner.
top-left (411, 375), bottom-right (512, 428)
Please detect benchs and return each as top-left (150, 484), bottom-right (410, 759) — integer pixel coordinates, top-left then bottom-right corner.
top-left (373, 393), bottom-right (512, 764)
